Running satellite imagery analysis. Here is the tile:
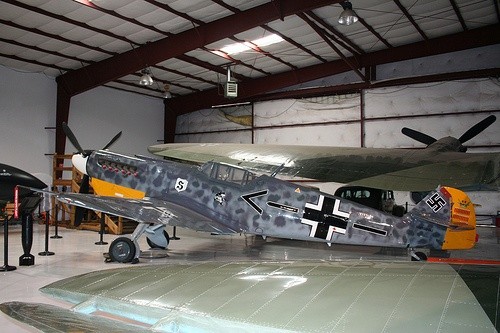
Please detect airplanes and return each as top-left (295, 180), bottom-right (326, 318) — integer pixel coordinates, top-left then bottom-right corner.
top-left (138, 114), bottom-right (500, 240)
top-left (15, 117), bottom-right (481, 264)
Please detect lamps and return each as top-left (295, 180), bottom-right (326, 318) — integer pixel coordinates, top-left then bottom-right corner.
top-left (338, 8), bottom-right (358, 25)
top-left (161, 92), bottom-right (172, 98)
top-left (137, 74), bottom-right (154, 86)
